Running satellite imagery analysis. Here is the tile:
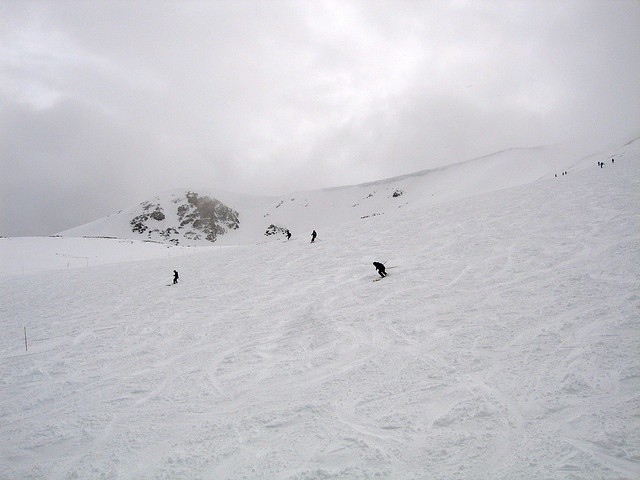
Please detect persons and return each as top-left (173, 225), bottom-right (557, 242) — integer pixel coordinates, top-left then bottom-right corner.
top-left (373, 261), bottom-right (388, 281)
top-left (285, 227), bottom-right (293, 243)
top-left (172, 267), bottom-right (180, 285)
top-left (309, 228), bottom-right (318, 243)
